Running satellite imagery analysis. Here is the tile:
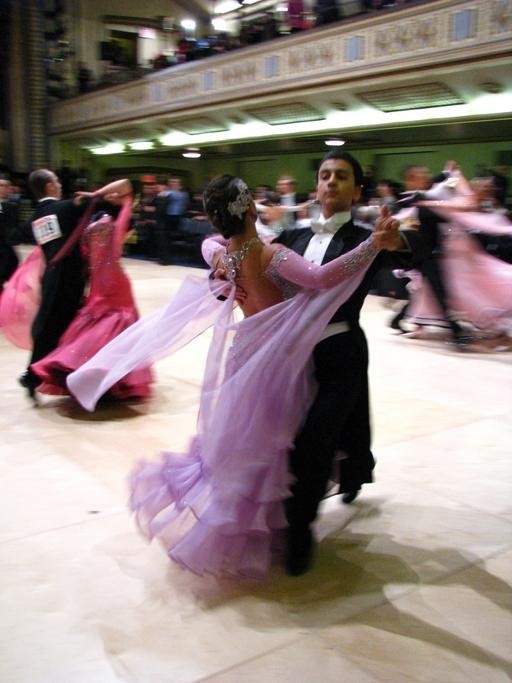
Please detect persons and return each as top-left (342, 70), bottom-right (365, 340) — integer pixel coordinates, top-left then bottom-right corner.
top-left (68, 170), bottom-right (403, 588)
top-left (396, 158), bottom-right (512, 344)
top-left (5, 166), bottom-right (130, 404)
top-left (384, 159), bottom-right (482, 349)
top-left (206, 146), bottom-right (426, 577)
top-left (1, 176), bottom-right (158, 401)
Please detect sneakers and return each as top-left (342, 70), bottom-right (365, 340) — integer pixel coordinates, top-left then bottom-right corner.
top-left (284, 525), bottom-right (311, 575)
top-left (17, 368), bottom-right (38, 393)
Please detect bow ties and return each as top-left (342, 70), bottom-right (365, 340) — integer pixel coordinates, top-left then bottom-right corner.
top-left (308, 220), bottom-right (337, 234)
